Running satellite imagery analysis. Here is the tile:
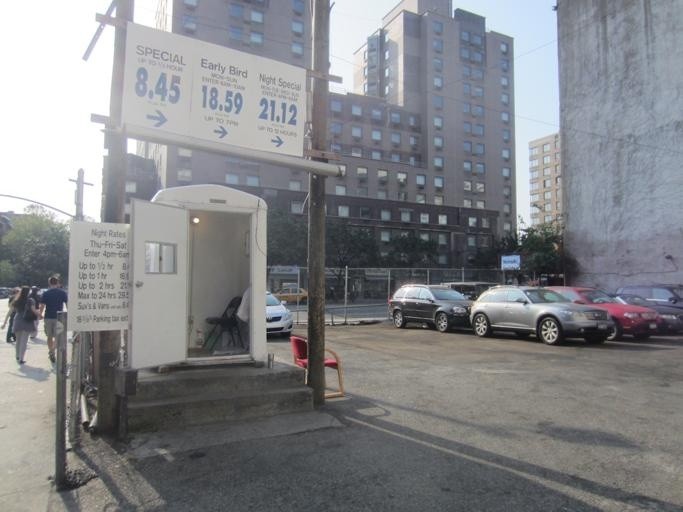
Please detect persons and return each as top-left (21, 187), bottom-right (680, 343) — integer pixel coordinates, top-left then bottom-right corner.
top-left (6, 286), bottom-right (21, 343)
top-left (0, 285), bottom-right (41, 364)
top-left (38, 277), bottom-right (68, 365)
top-left (28, 285), bottom-right (42, 340)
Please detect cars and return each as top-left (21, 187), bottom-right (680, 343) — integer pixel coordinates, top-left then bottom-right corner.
top-left (272, 287), bottom-right (308, 305)
top-left (0, 287), bottom-right (48, 301)
top-left (265, 291), bottom-right (292, 337)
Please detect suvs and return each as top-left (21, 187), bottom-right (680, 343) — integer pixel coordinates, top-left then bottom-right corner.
top-left (387, 275), bottom-right (682, 346)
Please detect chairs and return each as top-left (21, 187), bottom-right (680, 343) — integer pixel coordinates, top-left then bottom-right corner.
top-left (289, 335), bottom-right (345, 399)
top-left (201, 296), bottom-right (244, 351)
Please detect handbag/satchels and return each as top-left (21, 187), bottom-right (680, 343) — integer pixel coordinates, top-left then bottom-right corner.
top-left (23, 298), bottom-right (38, 321)
